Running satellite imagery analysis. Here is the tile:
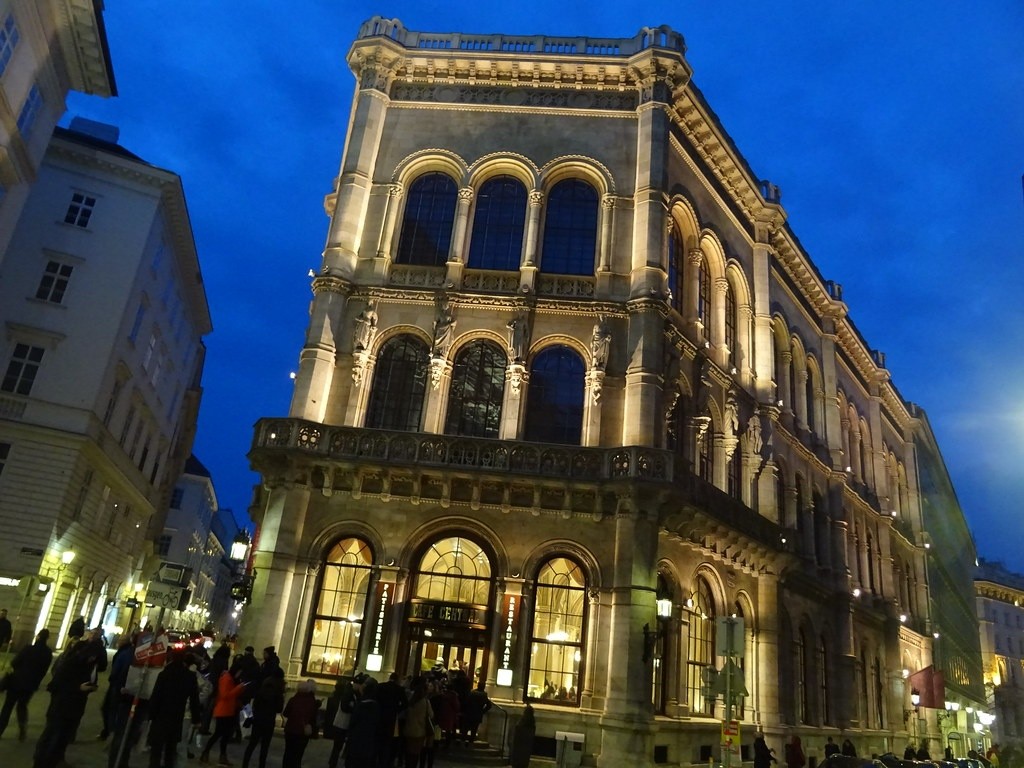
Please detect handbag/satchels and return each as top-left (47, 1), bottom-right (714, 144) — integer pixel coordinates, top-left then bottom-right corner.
top-left (243, 718), bottom-right (251, 729)
top-left (333, 700), bottom-right (351, 730)
top-left (305, 723), bottom-right (314, 736)
top-left (394, 713), bottom-right (399, 737)
top-left (0, 672), bottom-right (12, 690)
top-left (433, 724), bottom-right (442, 741)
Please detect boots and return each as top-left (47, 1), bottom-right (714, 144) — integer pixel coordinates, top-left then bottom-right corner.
top-left (198, 751), bottom-right (211, 766)
top-left (219, 755), bottom-right (234, 767)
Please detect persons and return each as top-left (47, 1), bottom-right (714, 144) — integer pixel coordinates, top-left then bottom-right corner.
top-left (697, 365), bottom-right (713, 416)
top-left (749, 410), bottom-right (763, 453)
top-left (591, 314), bottom-right (613, 371)
top-left (431, 302), bottom-right (457, 360)
top-left (353, 297), bottom-right (380, 350)
top-left (753, 731), bottom-right (1024, 768)
top-left (725, 390), bottom-right (739, 436)
top-left (0, 609), bottom-right (492, 768)
top-left (538, 681), bottom-right (577, 701)
top-left (667, 335), bottom-right (685, 388)
top-left (505, 308), bottom-right (530, 362)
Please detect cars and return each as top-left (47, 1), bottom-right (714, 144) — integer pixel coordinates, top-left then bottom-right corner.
top-left (166, 630), bottom-right (203, 650)
top-left (816, 753), bottom-right (986, 768)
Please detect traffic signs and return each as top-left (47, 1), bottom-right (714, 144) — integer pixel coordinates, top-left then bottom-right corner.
top-left (153, 561), bottom-right (195, 592)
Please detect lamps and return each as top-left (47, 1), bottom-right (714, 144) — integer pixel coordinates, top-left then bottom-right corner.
top-left (903, 685), bottom-right (920, 724)
top-left (936, 702), bottom-right (999, 734)
top-left (642, 581), bottom-right (673, 664)
top-left (223, 526), bottom-right (256, 618)
top-left (126, 583), bottom-right (145, 608)
top-left (496, 668), bottom-right (513, 688)
top-left (984, 675), bottom-right (1001, 700)
top-left (365, 654), bottom-right (383, 672)
top-left (48, 549), bottom-right (75, 572)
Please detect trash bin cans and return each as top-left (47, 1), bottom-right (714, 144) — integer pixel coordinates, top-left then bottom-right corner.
top-left (555, 731), bottom-right (586, 768)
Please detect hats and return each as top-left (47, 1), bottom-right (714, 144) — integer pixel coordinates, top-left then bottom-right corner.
top-left (264, 646), bottom-right (275, 656)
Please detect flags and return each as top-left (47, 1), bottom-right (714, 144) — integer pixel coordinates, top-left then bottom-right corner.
top-left (908, 665), bottom-right (946, 710)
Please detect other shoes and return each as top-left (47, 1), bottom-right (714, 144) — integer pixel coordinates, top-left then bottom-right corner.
top-left (95, 733), bottom-right (106, 740)
top-left (19, 725), bottom-right (27, 741)
top-left (464, 740), bottom-right (470, 748)
